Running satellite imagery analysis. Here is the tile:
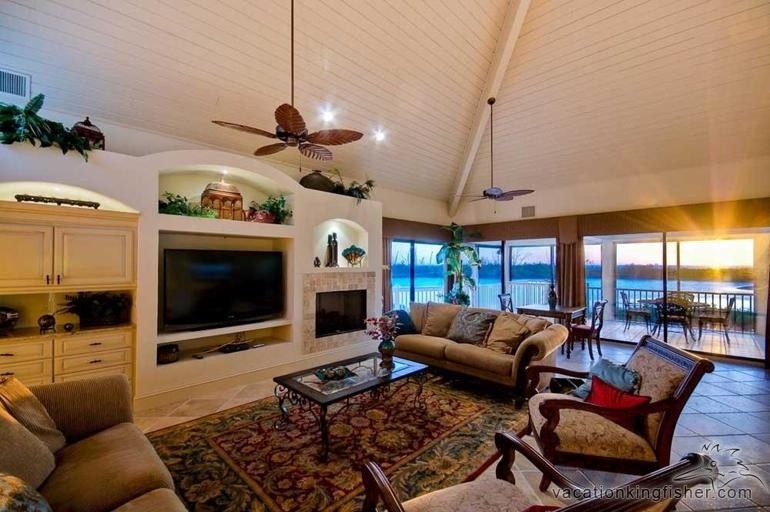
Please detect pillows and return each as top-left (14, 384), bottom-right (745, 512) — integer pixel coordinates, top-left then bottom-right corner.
top-left (0, 401), bottom-right (57, 493)
top-left (574, 358), bottom-right (640, 401)
top-left (500, 310), bottom-right (546, 335)
top-left (0, 470), bottom-right (53, 511)
top-left (446, 308), bottom-right (496, 349)
top-left (1, 374), bottom-right (67, 456)
top-left (486, 312), bottom-right (528, 355)
top-left (583, 375), bottom-right (651, 438)
top-left (382, 310), bottom-right (416, 336)
top-left (422, 301), bottom-right (463, 338)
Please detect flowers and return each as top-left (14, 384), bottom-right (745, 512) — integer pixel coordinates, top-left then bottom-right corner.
top-left (363, 315), bottom-right (401, 340)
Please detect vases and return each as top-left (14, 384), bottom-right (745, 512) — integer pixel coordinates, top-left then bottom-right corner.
top-left (378, 340), bottom-right (396, 374)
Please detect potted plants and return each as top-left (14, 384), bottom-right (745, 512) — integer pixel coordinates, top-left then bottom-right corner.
top-left (53, 291), bottom-right (131, 329)
top-left (437, 221), bottom-right (483, 307)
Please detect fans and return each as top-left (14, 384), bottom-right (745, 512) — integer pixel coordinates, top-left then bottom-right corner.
top-left (458, 96), bottom-right (535, 203)
top-left (212, 0), bottom-right (363, 162)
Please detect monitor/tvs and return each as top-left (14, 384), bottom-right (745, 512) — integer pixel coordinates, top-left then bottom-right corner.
top-left (162, 250), bottom-right (284, 333)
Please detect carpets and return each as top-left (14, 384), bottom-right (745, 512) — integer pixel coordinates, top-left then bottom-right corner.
top-left (144, 358), bottom-right (588, 512)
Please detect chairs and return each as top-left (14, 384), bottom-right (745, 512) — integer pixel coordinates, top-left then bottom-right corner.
top-left (498, 293), bottom-right (514, 314)
top-left (698, 297), bottom-right (735, 344)
top-left (657, 301), bottom-right (689, 345)
top-left (620, 290), bottom-right (651, 333)
top-left (569, 299), bottom-right (608, 360)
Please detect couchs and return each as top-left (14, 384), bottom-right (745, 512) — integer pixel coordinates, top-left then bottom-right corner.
top-left (358, 430), bottom-right (717, 512)
top-left (526, 334), bottom-right (715, 494)
top-left (0, 370), bottom-right (195, 511)
top-left (381, 301), bottom-right (570, 410)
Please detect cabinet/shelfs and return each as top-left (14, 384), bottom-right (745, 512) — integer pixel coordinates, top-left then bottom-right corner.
top-left (0, 325), bottom-right (136, 426)
top-left (0, 200), bottom-right (139, 289)
